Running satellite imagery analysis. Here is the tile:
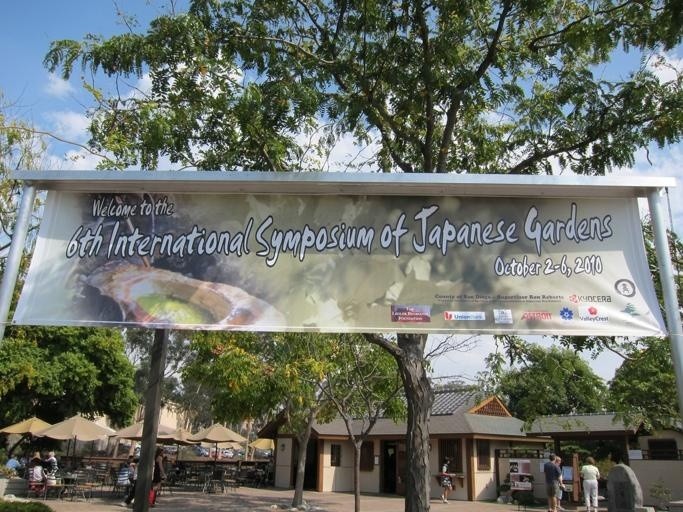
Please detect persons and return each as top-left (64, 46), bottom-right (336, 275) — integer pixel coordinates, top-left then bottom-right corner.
top-left (120, 461), bottom-right (139, 507)
top-left (555, 456), bottom-right (564, 510)
top-left (5, 450), bottom-right (77, 494)
top-left (266, 456), bottom-right (274, 487)
top-left (578, 456), bottom-right (600, 512)
top-left (544, 453), bottom-right (565, 512)
top-left (127, 455), bottom-right (133, 473)
top-left (118, 464), bottom-right (130, 485)
top-left (155, 448), bottom-right (167, 495)
top-left (439, 456), bottom-right (451, 503)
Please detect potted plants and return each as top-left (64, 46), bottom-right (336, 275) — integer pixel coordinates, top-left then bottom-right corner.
top-left (0, 464), bottom-right (26, 497)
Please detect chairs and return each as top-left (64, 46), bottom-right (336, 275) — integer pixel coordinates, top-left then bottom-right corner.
top-left (26, 459), bottom-right (274, 503)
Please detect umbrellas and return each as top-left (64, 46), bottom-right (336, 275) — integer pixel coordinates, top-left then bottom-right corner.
top-left (115, 419), bottom-right (174, 442)
top-left (0, 415), bottom-right (51, 477)
top-left (248, 438), bottom-right (275, 450)
top-left (198, 441), bottom-right (242, 449)
top-left (156, 426), bottom-right (199, 447)
top-left (187, 421), bottom-right (246, 442)
top-left (37, 413), bottom-right (114, 473)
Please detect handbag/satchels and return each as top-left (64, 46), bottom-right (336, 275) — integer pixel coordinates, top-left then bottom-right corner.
top-left (46, 473), bottom-right (57, 486)
top-left (149, 486), bottom-right (158, 506)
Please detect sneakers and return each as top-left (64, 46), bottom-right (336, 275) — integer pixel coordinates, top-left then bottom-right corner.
top-left (120, 501), bottom-right (128, 507)
top-left (439, 494), bottom-right (449, 504)
top-left (548, 506), bottom-right (566, 512)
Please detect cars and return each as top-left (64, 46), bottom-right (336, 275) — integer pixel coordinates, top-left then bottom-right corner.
top-left (123, 441), bottom-right (272, 459)
